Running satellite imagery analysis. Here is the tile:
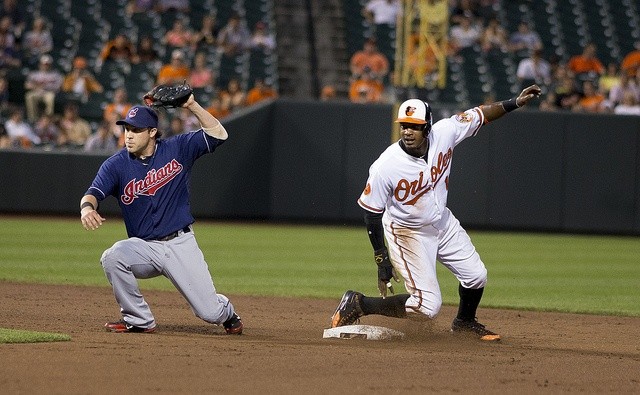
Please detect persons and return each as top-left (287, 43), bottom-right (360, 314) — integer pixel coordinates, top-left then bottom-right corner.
top-left (518, 41), bottom-right (550, 91)
top-left (450, 0), bottom-right (506, 58)
top-left (509, 22), bottom-right (543, 58)
top-left (550, 44), bottom-right (640, 114)
top-left (79, 92), bottom-right (247, 336)
top-left (207, 79), bottom-right (274, 122)
top-left (328, 82), bottom-right (544, 343)
top-left (99, 1), bottom-right (280, 92)
top-left (104, 88), bottom-right (200, 149)
top-left (349, 38), bottom-right (389, 102)
top-left (363, 0), bottom-right (398, 49)
top-left (1, 1), bottom-right (103, 147)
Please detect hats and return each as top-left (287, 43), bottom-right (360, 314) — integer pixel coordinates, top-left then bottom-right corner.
top-left (115, 106), bottom-right (160, 128)
top-left (393, 99), bottom-right (433, 139)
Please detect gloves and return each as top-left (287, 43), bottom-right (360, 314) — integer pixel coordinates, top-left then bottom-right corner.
top-left (375, 247), bottom-right (393, 282)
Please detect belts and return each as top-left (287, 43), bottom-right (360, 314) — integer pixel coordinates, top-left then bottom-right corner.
top-left (156, 227), bottom-right (191, 241)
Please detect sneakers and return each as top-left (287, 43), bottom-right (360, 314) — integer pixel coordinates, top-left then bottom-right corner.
top-left (451, 317), bottom-right (501, 341)
top-left (331, 290), bottom-right (366, 327)
top-left (222, 312), bottom-right (243, 334)
top-left (105, 320), bottom-right (156, 334)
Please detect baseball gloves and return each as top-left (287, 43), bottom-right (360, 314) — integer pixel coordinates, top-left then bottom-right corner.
top-left (143, 79), bottom-right (194, 113)
